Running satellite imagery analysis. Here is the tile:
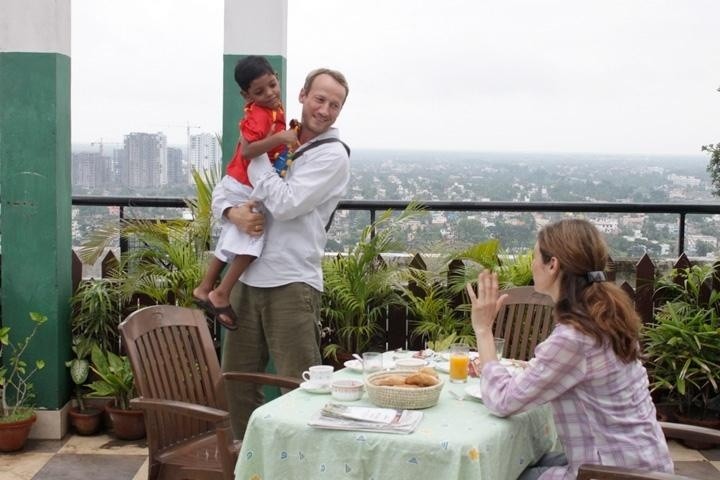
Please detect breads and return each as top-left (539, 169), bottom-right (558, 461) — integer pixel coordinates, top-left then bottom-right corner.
top-left (378, 367), bottom-right (438, 388)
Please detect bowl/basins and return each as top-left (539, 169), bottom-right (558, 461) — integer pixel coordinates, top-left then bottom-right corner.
top-left (331, 378), bottom-right (364, 404)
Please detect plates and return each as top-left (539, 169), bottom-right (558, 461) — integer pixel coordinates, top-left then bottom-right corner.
top-left (442, 350), bottom-right (480, 361)
top-left (299, 382), bottom-right (331, 396)
top-left (465, 381), bottom-right (482, 401)
top-left (438, 361), bottom-right (449, 373)
top-left (342, 360), bottom-right (392, 372)
top-left (394, 358), bottom-right (427, 368)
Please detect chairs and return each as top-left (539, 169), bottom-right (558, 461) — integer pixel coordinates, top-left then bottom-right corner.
top-left (577, 419), bottom-right (719, 480)
top-left (492, 287), bottom-right (556, 363)
top-left (116, 304), bottom-right (307, 480)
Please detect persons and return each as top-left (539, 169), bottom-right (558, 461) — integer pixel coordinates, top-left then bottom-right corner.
top-left (210, 67), bottom-right (350, 441)
top-left (191, 55), bottom-right (297, 330)
top-left (467, 217), bottom-right (674, 480)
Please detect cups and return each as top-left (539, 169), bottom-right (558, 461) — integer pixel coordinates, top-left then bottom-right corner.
top-left (301, 364), bottom-right (334, 387)
top-left (494, 337), bottom-right (505, 363)
top-left (449, 342), bottom-right (470, 384)
top-left (361, 352), bottom-right (383, 377)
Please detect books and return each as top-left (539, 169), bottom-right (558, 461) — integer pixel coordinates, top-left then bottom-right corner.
top-left (308, 401), bottom-right (422, 436)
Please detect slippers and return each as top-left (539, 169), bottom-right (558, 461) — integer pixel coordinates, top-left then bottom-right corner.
top-left (194, 300), bottom-right (239, 330)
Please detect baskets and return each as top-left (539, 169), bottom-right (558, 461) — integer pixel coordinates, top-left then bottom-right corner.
top-left (365, 369), bottom-right (444, 409)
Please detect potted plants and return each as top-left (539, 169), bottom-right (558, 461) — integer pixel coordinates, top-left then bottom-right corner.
top-left (0, 311), bottom-right (146, 453)
top-left (640, 262), bottom-right (720, 449)
